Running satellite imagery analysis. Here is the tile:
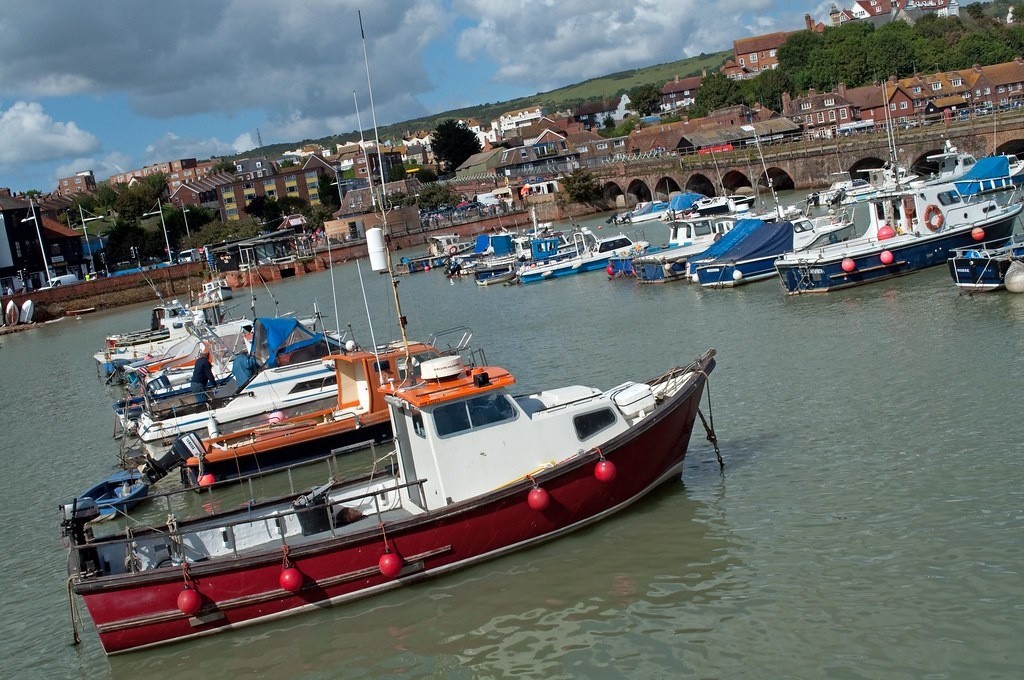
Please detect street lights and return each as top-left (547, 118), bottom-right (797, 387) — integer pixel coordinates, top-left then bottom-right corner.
top-left (181, 199), bottom-right (192, 241)
top-left (21, 198), bottom-right (54, 290)
top-left (142, 197), bottom-right (172, 264)
top-left (78, 204), bottom-right (105, 273)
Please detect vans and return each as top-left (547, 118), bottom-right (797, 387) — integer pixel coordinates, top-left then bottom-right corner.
top-left (37, 275), bottom-right (78, 291)
top-left (177, 248), bottom-right (199, 266)
top-left (476, 188), bottom-right (514, 214)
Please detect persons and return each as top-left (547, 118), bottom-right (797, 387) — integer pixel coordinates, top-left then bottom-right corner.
top-left (312, 227), bottom-right (323, 245)
top-left (26, 275), bottom-right (37, 292)
top-left (56, 282), bottom-right (60, 287)
top-left (423, 212), bottom-right (448, 226)
top-left (6, 286), bottom-right (14, 296)
top-left (488, 200), bottom-right (516, 214)
top-left (142, 254), bottom-right (162, 265)
top-left (401, 256), bottom-right (410, 272)
top-left (84, 270), bottom-right (106, 281)
top-left (191, 350), bottom-right (217, 407)
top-left (521, 183), bottom-right (530, 209)
top-left (164, 243), bottom-right (205, 264)
top-left (232, 348), bottom-right (256, 389)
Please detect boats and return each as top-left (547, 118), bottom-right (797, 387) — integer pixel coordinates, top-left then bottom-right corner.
top-left (391, 81), bottom-right (1023, 296)
top-left (57, 8), bottom-right (721, 657)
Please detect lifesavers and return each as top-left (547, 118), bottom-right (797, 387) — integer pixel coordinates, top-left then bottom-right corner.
top-left (107, 340), bottom-right (118, 346)
top-left (924, 204), bottom-right (944, 231)
top-left (449, 246), bottom-right (457, 255)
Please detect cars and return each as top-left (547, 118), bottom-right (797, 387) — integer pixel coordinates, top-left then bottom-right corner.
top-left (421, 202), bottom-right (488, 228)
top-left (951, 100), bottom-right (1022, 120)
top-left (891, 119), bottom-right (931, 131)
